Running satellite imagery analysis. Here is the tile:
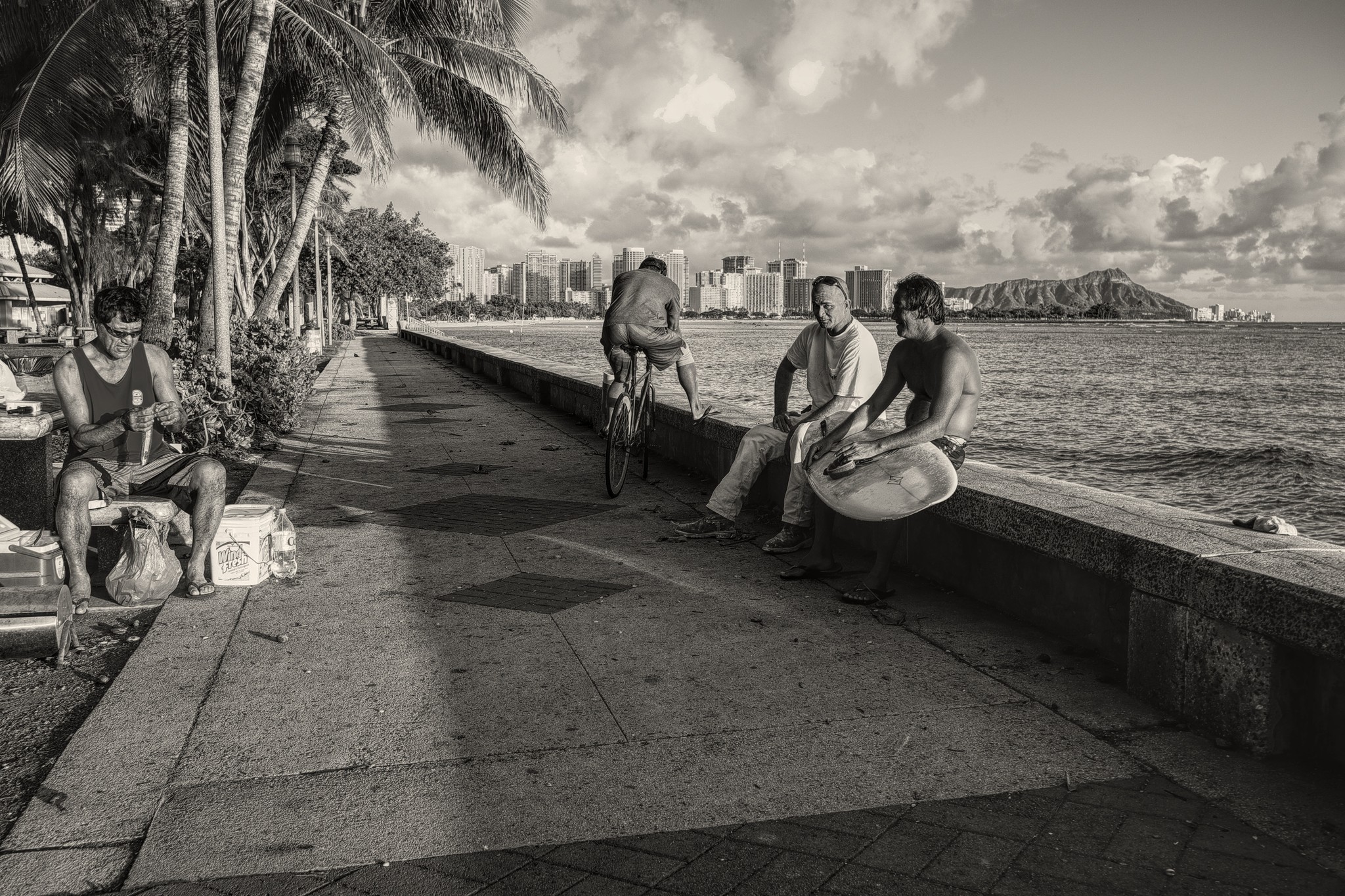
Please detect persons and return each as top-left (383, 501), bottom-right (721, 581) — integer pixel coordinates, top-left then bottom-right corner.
top-left (774, 271), bottom-right (980, 605)
top-left (52, 286), bottom-right (227, 616)
top-left (673, 275), bottom-right (884, 552)
top-left (599, 257), bottom-right (721, 439)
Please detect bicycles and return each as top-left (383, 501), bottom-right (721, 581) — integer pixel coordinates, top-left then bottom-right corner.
top-left (604, 344), bottom-right (656, 497)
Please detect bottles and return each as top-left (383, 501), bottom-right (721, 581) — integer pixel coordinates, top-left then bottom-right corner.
top-left (271, 508), bottom-right (297, 579)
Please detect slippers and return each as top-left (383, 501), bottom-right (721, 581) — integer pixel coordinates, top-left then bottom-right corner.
top-left (779, 563), bottom-right (843, 579)
top-left (598, 428), bottom-right (613, 437)
top-left (64, 598), bottom-right (91, 618)
top-left (186, 577), bottom-right (217, 598)
top-left (692, 405), bottom-right (722, 427)
top-left (840, 584), bottom-right (897, 605)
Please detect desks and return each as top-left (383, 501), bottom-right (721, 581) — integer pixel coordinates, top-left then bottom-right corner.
top-left (357, 317), bottom-right (378, 329)
top-left (0, 326), bottom-right (32, 344)
top-left (76, 327), bottom-right (95, 344)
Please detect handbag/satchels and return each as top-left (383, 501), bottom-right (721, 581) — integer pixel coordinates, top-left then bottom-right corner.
top-left (105, 513), bottom-right (184, 607)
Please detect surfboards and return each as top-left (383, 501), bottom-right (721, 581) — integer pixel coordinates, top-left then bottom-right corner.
top-left (800, 410), bottom-right (959, 522)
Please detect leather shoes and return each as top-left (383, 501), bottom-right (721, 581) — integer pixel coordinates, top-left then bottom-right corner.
top-left (674, 509), bottom-right (736, 539)
top-left (761, 522), bottom-right (815, 553)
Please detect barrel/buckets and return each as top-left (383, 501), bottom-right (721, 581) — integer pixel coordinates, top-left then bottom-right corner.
top-left (210, 503), bottom-right (275, 585)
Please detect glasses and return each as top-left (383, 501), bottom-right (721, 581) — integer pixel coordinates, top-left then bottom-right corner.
top-left (99, 321), bottom-right (144, 338)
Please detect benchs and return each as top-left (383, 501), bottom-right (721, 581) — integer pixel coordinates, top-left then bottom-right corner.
top-left (61, 335), bottom-right (83, 348)
top-left (356, 323), bottom-right (379, 329)
top-left (86, 491), bottom-right (191, 574)
top-left (24, 334), bottom-right (43, 343)
